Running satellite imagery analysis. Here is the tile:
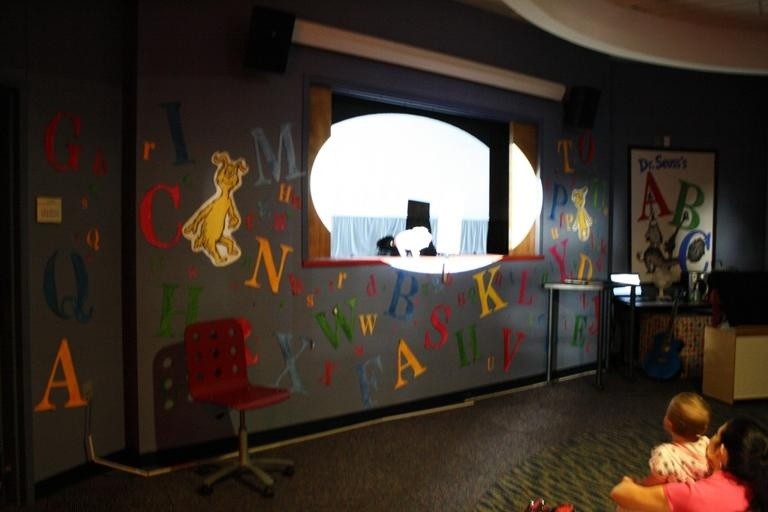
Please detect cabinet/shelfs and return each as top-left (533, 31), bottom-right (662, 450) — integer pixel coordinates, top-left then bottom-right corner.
top-left (703, 323), bottom-right (767, 407)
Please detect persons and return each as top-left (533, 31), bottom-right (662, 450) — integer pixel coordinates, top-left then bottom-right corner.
top-left (647, 390), bottom-right (712, 484)
top-left (607, 413), bottom-right (768, 512)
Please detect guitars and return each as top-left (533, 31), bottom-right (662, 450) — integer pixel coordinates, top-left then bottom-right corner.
top-left (644, 287), bottom-right (684, 379)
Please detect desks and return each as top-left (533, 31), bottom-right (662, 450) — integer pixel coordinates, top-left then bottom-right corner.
top-left (618, 292), bottom-right (718, 376)
top-left (543, 279), bottom-right (613, 392)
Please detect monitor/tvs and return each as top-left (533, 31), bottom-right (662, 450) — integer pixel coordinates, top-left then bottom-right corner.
top-left (611, 273), bottom-right (642, 297)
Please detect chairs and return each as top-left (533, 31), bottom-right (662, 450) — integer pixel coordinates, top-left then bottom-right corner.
top-left (183, 318), bottom-right (297, 496)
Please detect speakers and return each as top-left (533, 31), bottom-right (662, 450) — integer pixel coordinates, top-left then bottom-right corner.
top-left (244, 4), bottom-right (296, 72)
top-left (561, 85), bottom-right (600, 135)
